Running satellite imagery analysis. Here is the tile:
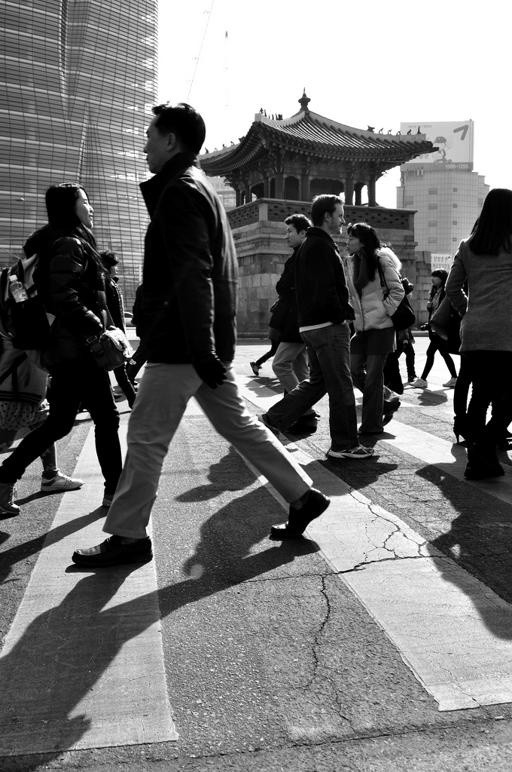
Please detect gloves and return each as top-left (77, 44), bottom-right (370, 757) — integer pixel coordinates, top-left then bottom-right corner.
top-left (191, 350), bottom-right (226, 389)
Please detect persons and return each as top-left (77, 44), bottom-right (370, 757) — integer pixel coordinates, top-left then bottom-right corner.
top-left (0, 181), bottom-right (122, 517)
top-left (408, 190), bottom-right (512, 480)
top-left (99, 250), bottom-right (144, 407)
top-left (1, 330), bottom-right (84, 492)
top-left (70, 104), bottom-right (331, 567)
top-left (250, 194), bottom-right (416, 459)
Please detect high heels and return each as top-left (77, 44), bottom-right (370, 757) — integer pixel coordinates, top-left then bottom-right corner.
top-left (453, 416), bottom-right (467, 443)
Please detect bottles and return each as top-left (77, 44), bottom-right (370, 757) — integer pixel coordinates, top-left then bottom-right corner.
top-left (9, 274), bottom-right (29, 303)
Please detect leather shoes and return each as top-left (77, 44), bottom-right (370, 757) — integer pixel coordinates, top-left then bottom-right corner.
top-left (272, 488), bottom-right (330, 535)
top-left (383, 401), bottom-right (401, 425)
top-left (72, 534), bottom-right (152, 567)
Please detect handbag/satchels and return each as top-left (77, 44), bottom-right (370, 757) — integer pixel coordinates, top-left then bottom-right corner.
top-left (384, 292), bottom-right (415, 332)
top-left (99, 325), bottom-right (135, 370)
top-left (430, 296), bottom-right (462, 342)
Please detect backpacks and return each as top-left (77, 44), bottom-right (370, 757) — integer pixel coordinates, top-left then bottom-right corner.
top-left (0, 238), bottom-right (88, 350)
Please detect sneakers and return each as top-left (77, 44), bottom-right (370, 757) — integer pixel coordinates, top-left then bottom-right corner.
top-left (250, 362), bottom-right (259, 375)
top-left (414, 379), bottom-right (428, 388)
top-left (0, 482), bottom-right (20, 514)
top-left (327, 444), bottom-right (375, 459)
top-left (40, 472), bottom-right (84, 492)
top-left (443, 377), bottom-right (457, 386)
